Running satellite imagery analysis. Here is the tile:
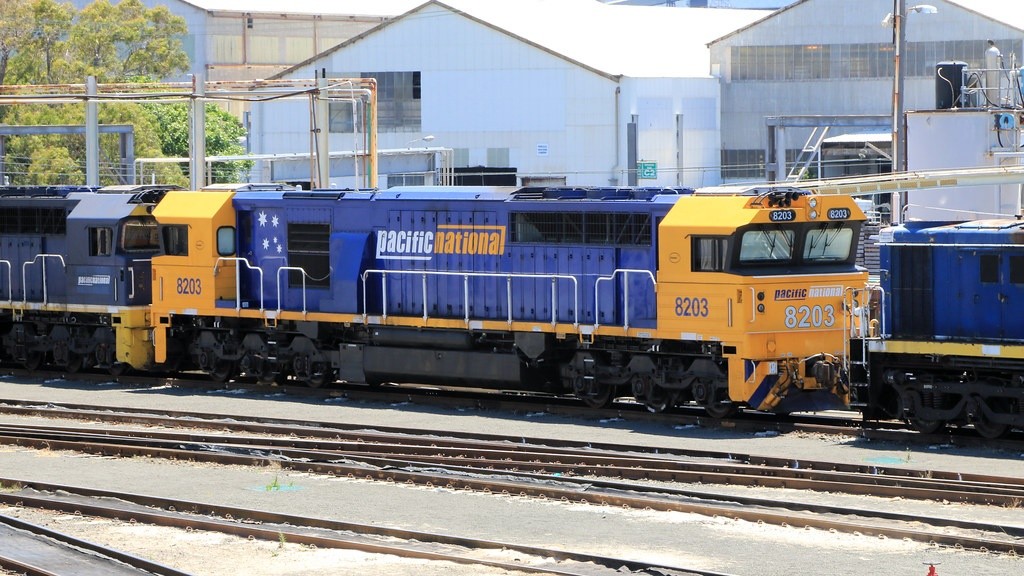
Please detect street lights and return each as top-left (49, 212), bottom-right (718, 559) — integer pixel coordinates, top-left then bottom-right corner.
top-left (879, 0), bottom-right (941, 225)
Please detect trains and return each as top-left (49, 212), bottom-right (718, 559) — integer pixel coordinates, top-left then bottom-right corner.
top-left (0, 165), bottom-right (1024, 444)
